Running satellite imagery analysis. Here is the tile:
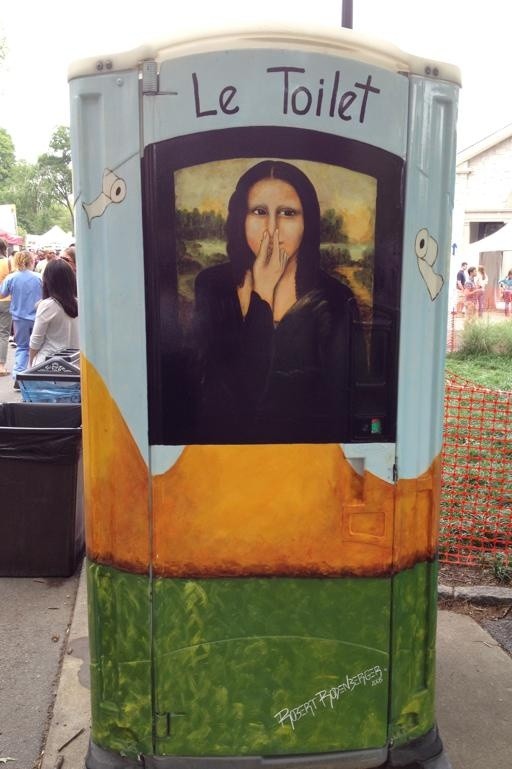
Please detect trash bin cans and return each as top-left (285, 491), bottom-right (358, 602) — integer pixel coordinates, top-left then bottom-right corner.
top-left (0, 403), bottom-right (86, 578)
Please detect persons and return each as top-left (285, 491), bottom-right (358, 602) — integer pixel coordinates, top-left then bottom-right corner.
top-left (454, 261), bottom-right (469, 318)
top-left (498, 269), bottom-right (512, 319)
top-left (463, 266), bottom-right (483, 320)
top-left (0, 239), bottom-right (79, 394)
top-left (473, 265), bottom-right (488, 317)
top-left (185, 160), bottom-right (371, 428)
